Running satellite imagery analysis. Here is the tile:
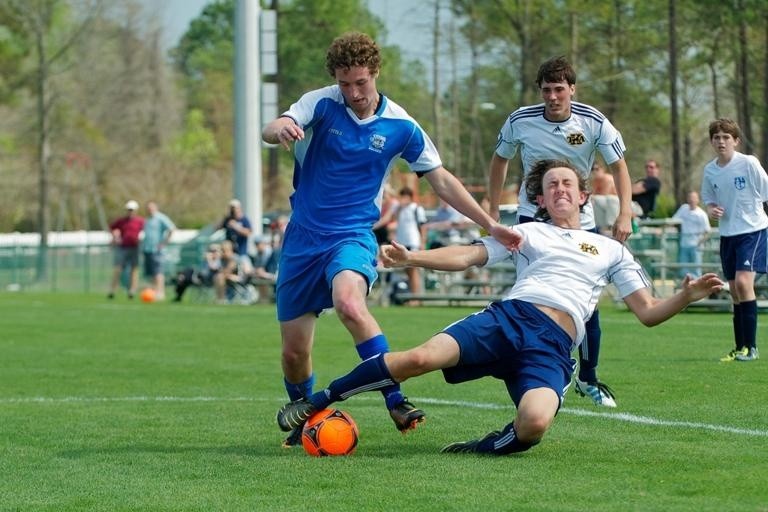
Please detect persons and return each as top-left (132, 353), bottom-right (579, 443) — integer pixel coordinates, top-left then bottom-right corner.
top-left (673, 191), bottom-right (710, 289)
top-left (107, 201), bottom-right (176, 301)
top-left (699, 118), bottom-right (767, 363)
top-left (590, 159), bottom-right (661, 237)
top-left (259, 29), bottom-right (526, 450)
top-left (373, 186), bottom-right (490, 306)
top-left (487, 56), bottom-right (633, 411)
top-left (175, 200), bottom-right (286, 305)
top-left (276, 158), bottom-right (723, 457)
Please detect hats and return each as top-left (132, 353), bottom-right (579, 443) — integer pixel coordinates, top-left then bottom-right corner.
top-left (127, 200), bottom-right (138, 210)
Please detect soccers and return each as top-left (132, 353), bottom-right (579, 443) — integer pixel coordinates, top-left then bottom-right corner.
top-left (141, 289), bottom-right (154, 303)
top-left (302, 409), bottom-right (358, 457)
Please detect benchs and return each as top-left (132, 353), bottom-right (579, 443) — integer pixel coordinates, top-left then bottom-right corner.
top-left (395, 218), bottom-right (767, 308)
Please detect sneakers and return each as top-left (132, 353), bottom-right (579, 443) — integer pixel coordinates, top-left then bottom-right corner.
top-left (735, 347), bottom-right (759, 361)
top-left (389, 398), bottom-right (425, 433)
top-left (281, 427), bottom-right (302, 447)
top-left (574, 377), bottom-right (618, 407)
top-left (719, 346), bottom-right (748, 362)
top-left (277, 398), bottom-right (319, 432)
top-left (439, 441), bottom-right (477, 455)
